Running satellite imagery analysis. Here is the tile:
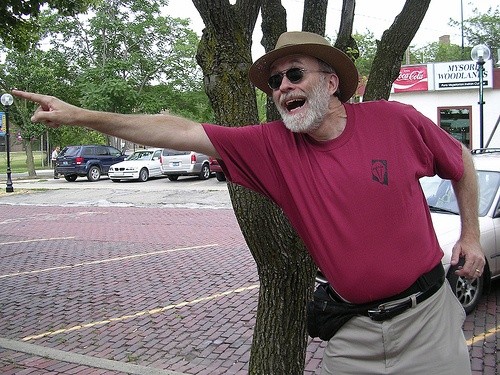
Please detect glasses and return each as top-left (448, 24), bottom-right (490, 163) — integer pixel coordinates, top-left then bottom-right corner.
top-left (266, 67), bottom-right (335, 90)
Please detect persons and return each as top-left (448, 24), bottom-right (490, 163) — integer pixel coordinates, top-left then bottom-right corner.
top-left (12, 32), bottom-right (486, 375)
top-left (52, 147), bottom-right (60, 179)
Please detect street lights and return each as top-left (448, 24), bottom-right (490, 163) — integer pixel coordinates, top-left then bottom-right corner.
top-left (470, 44), bottom-right (492, 150)
top-left (1, 93), bottom-right (15, 193)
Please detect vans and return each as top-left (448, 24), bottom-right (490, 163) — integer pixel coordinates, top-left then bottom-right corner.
top-left (160, 147), bottom-right (211, 181)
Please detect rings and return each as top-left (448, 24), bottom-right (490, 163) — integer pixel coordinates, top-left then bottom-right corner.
top-left (475, 268), bottom-right (480, 273)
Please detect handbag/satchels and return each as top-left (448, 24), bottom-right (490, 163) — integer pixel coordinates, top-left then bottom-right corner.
top-left (305, 283), bottom-right (360, 341)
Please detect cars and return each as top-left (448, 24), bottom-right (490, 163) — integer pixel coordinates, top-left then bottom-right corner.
top-left (419, 149), bottom-right (500, 313)
top-left (108, 148), bottom-right (162, 182)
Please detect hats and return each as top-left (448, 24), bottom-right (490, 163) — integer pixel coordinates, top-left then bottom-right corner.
top-left (247, 30), bottom-right (360, 103)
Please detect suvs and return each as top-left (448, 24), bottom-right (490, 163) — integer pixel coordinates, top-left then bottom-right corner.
top-left (55, 145), bottom-right (127, 182)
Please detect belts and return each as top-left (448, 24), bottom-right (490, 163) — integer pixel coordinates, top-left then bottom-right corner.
top-left (358, 277), bottom-right (446, 321)
